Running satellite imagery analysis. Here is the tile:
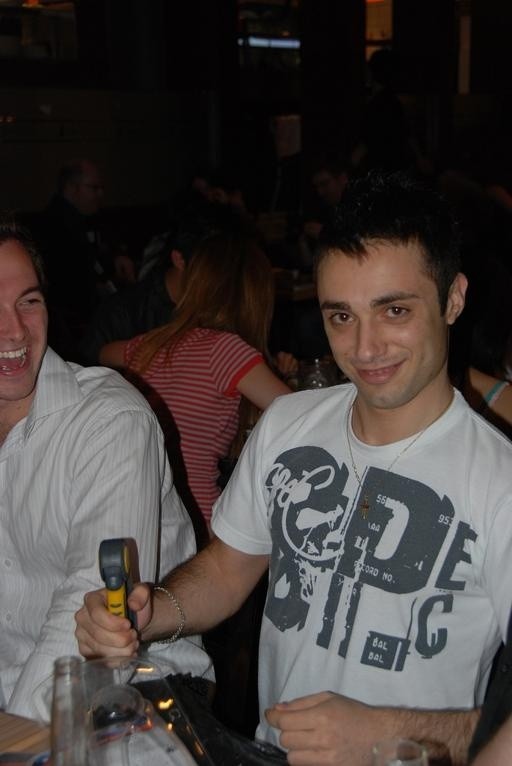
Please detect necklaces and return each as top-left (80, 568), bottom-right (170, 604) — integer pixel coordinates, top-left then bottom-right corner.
top-left (346, 395), bottom-right (427, 519)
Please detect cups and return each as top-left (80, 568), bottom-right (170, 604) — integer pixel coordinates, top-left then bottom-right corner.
top-left (301, 360), bottom-right (332, 389)
top-left (372, 739), bottom-right (429, 766)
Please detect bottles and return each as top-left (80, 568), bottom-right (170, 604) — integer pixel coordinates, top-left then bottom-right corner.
top-left (48, 655), bottom-right (85, 766)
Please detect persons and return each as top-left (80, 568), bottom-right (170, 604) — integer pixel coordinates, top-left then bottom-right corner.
top-left (79, 217), bottom-right (300, 381)
top-left (93, 214), bottom-right (298, 554)
top-left (1, 226), bottom-right (225, 733)
top-left (448, 360), bottom-right (509, 438)
top-left (72, 210), bottom-right (510, 766)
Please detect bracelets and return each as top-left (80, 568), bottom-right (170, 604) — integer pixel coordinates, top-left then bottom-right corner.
top-left (477, 381), bottom-right (510, 415)
top-left (151, 585), bottom-right (186, 646)
